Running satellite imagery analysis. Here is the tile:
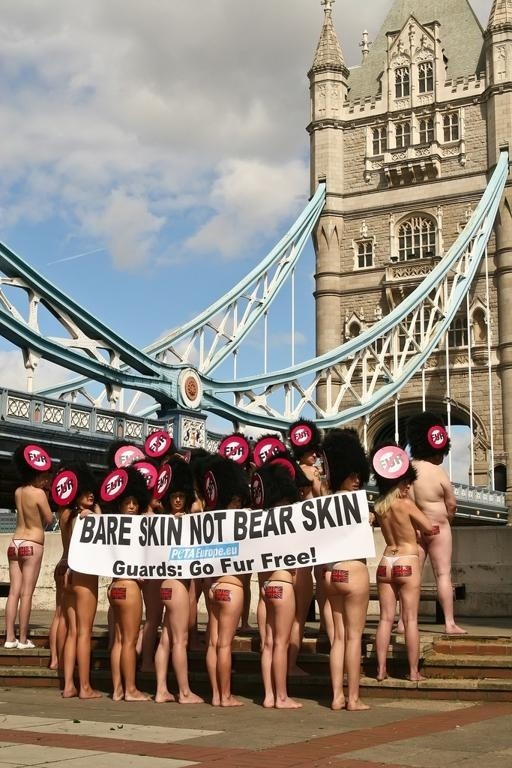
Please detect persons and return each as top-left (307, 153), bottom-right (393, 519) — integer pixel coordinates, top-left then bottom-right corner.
top-left (4, 412), bottom-right (470, 710)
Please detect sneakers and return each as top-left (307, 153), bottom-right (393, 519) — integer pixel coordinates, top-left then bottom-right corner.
top-left (4, 639), bottom-right (18, 647)
top-left (17, 639), bottom-right (35, 648)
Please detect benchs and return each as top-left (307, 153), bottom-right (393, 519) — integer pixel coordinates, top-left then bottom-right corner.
top-left (305, 580), bottom-right (470, 625)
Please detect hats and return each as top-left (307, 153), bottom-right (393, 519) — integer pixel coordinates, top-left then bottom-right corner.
top-left (13, 418), bottom-right (447, 514)
